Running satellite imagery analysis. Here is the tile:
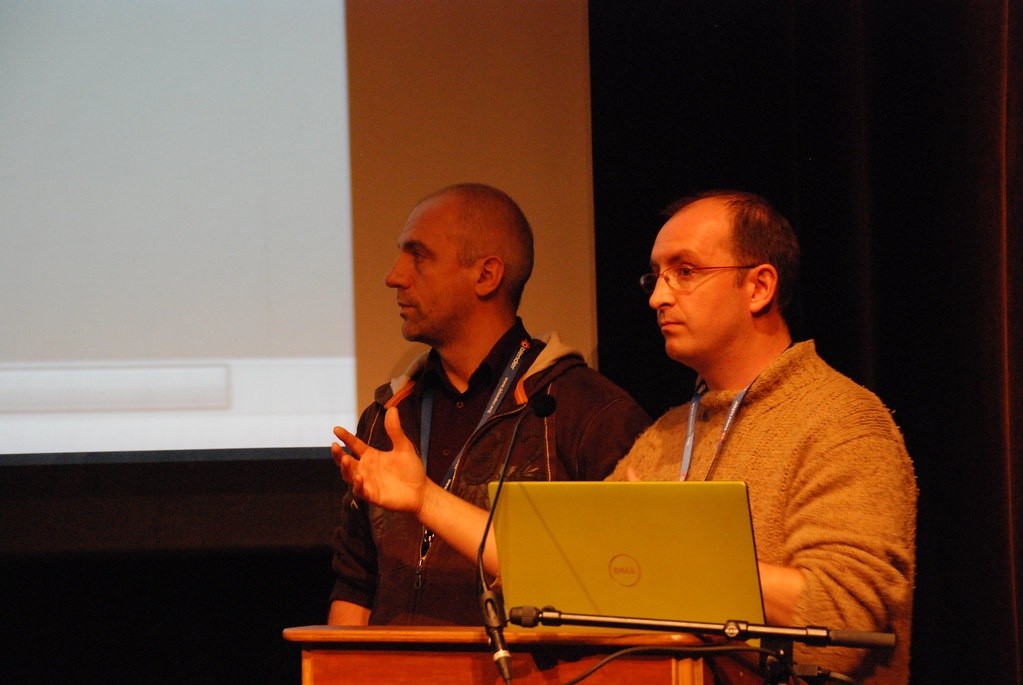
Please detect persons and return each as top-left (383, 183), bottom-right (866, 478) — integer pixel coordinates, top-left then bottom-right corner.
top-left (331, 190), bottom-right (919, 685)
top-left (324, 183), bottom-right (653, 626)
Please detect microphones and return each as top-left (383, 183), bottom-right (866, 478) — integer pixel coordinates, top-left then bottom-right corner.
top-left (475, 394), bottom-right (557, 685)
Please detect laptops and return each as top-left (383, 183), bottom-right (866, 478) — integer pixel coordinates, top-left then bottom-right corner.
top-left (484, 480), bottom-right (766, 629)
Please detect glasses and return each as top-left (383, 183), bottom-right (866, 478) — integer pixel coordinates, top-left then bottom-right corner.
top-left (640, 264), bottom-right (759, 295)
